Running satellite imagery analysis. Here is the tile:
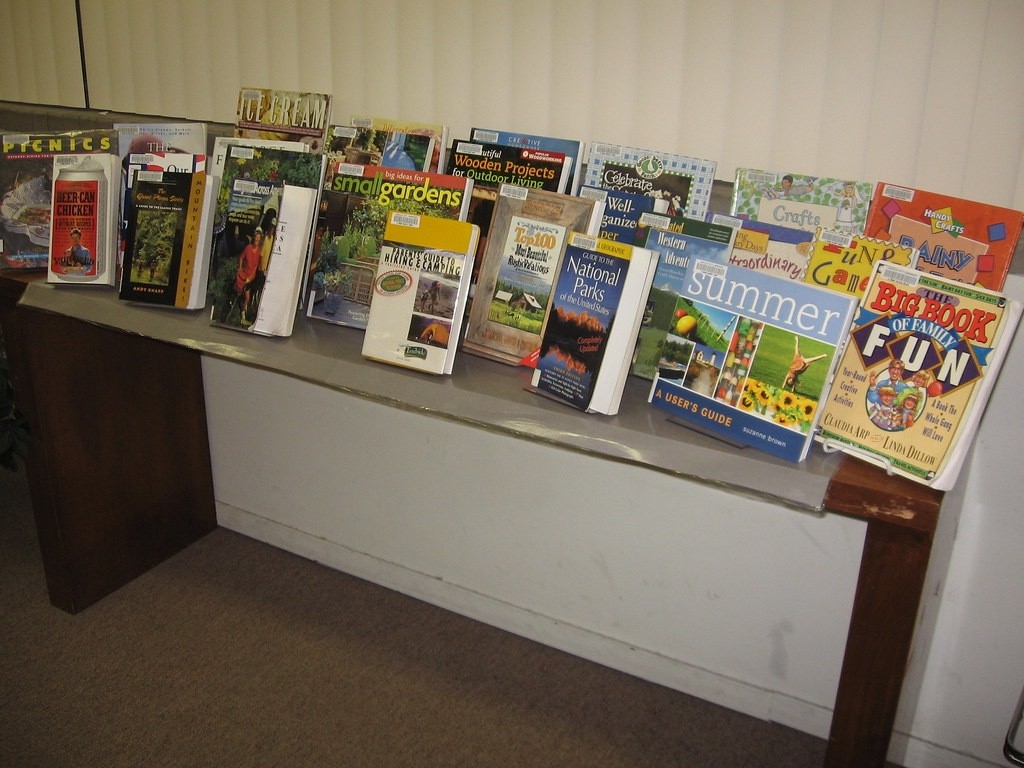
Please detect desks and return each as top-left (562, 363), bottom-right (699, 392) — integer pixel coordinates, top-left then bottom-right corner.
top-left (0, 269), bottom-right (971, 768)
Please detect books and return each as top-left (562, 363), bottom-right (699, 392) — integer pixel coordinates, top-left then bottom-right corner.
top-left (0, 86), bottom-right (1024, 493)
